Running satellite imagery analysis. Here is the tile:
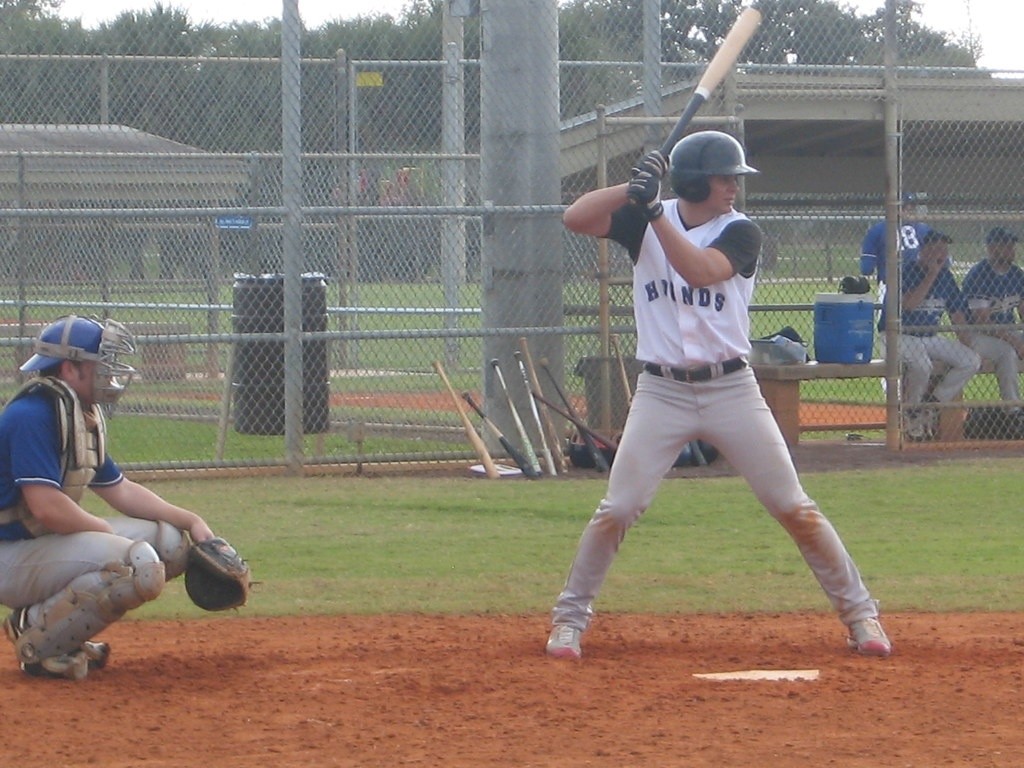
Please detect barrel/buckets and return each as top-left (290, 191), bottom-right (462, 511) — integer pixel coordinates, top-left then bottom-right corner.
top-left (229, 274), bottom-right (325, 436)
top-left (814, 291), bottom-right (879, 364)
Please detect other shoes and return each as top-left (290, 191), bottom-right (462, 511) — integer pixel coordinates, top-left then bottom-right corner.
top-left (922, 405), bottom-right (934, 439)
top-left (1005, 404), bottom-right (1024, 416)
top-left (900, 409), bottom-right (925, 441)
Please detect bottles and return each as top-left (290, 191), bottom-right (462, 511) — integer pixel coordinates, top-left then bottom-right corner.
top-left (1011, 305), bottom-right (1021, 325)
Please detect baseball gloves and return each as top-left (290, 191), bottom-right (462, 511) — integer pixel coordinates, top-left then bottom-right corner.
top-left (185, 538), bottom-right (251, 611)
top-left (841, 277), bottom-right (871, 294)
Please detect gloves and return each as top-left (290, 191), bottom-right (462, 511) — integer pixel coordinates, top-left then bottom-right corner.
top-left (630, 150), bottom-right (671, 179)
top-left (626, 172), bottom-right (664, 222)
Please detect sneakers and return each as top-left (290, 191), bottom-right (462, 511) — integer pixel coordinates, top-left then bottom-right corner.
top-left (845, 616), bottom-right (891, 655)
top-left (4, 604), bottom-right (43, 678)
top-left (546, 624), bottom-right (582, 661)
top-left (68, 639), bottom-right (110, 669)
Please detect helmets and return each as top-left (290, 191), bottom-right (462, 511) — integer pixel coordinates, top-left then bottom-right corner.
top-left (670, 130), bottom-right (760, 202)
top-left (896, 191), bottom-right (916, 205)
top-left (20, 311), bottom-right (104, 370)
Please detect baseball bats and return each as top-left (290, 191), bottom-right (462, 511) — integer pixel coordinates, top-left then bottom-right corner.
top-left (461, 390), bottom-right (538, 476)
top-left (627, 8), bottom-right (762, 209)
top-left (691, 440), bottom-right (707, 467)
top-left (514, 351), bottom-right (558, 476)
top-left (491, 359), bottom-right (541, 473)
top-left (215, 341), bottom-right (235, 460)
top-left (540, 358), bottom-right (609, 473)
top-left (532, 391), bottom-right (617, 452)
top-left (432, 361), bottom-right (500, 479)
top-left (612, 333), bottom-right (632, 407)
top-left (520, 337), bottom-right (568, 474)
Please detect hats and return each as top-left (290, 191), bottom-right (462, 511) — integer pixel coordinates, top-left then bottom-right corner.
top-left (922, 230), bottom-right (953, 244)
top-left (985, 227), bottom-right (1018, 243)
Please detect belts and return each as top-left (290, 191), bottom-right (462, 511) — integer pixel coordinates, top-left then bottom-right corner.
top-left (644, 355), bottom-right (747, 381)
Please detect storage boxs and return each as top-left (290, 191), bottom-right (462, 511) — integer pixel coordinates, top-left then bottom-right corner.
top-left (814, 291), bottom-right (873, 363)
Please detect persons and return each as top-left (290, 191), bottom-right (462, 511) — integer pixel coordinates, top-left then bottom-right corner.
top-left (545, 130), bottom-right (891, 657)
top-left (859, 191), bottom-right (1024, 439)
top-left (0, 315), bottom-right (217, 679)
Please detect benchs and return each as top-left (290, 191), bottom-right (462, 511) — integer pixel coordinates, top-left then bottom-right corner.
top-left (748, 359), bottom-right (1024, 443)
top-left (0, 322), bottom-right (190, 381)
